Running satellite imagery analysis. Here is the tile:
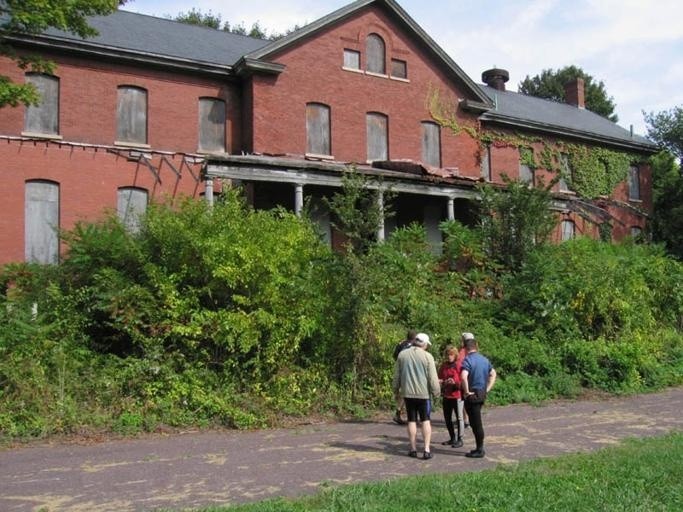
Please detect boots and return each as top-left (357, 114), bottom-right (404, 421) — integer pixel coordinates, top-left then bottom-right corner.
top-left (394, 409), bottom-right (404, 424)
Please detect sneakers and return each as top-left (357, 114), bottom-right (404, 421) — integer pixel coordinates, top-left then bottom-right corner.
top-left (424, 451), bottom-right (433, 460)
top-left (408, 450), bottom-right (417, 457)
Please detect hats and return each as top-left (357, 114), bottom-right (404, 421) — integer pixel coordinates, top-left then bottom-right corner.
top-left (464, 333), bottom-right (474, 340)
top-left (414, 332), bottom-right (432, 346)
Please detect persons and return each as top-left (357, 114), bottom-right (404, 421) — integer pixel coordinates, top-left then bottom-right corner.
top-left (391, 329), bottom-right (430, 423)
top-left (459, 338), bottom-right (497, 457)
top-left (391, 332), bottom-right (444, 458)
top-left (455, 331), bottom-right (480, 430)
top-left (436, 344), bottom-right (464, 448)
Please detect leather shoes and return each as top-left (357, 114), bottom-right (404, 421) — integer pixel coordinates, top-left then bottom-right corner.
top-left (466, 449), bottom-right (485, 458)
top-left (443, 441), bottom-right (463, 448)
